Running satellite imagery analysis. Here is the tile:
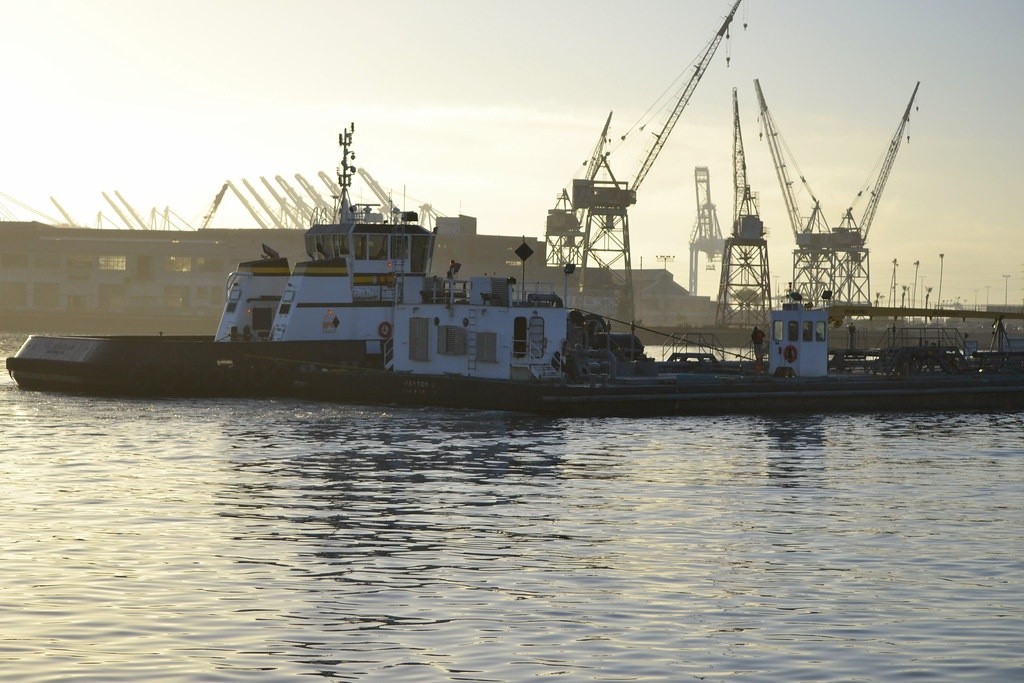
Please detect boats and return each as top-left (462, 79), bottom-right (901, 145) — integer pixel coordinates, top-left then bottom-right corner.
top-left (0, 122), bottom-right (1024, 417)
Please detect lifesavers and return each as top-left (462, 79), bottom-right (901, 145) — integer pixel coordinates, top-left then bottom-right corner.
top-left (379, 320), bottom-right (392, 337)
top-left (784, 345), bottom-right (797, 362)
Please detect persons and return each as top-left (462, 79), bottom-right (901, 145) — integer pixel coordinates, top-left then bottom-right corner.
top-left (750, 327), bottom-right (766, 362)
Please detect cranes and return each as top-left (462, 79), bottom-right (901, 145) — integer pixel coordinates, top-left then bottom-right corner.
top-left (544, 1), bottom-right (923, 355)
